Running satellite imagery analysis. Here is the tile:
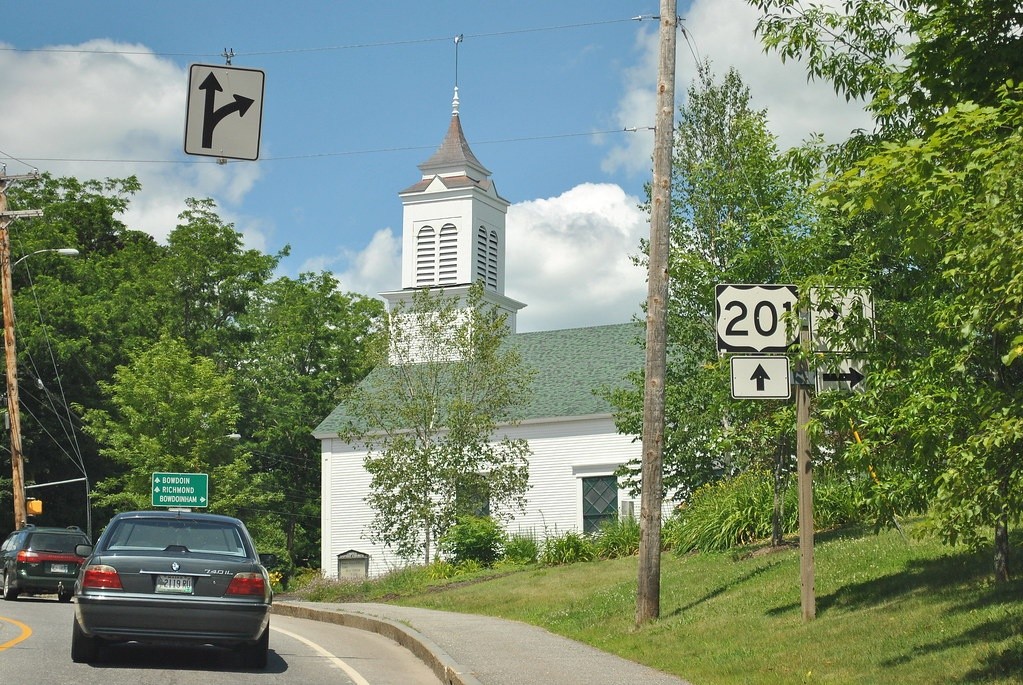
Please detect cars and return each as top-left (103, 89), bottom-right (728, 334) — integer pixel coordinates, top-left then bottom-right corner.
top-left (69, 510), bottom-right (278, 668)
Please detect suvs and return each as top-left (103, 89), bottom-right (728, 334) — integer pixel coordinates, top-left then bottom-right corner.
top-left (1, 524), bottom-right (94, 603)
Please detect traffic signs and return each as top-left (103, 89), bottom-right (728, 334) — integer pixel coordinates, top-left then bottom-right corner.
top-left (151, 472), bottom-right (208, 508)
